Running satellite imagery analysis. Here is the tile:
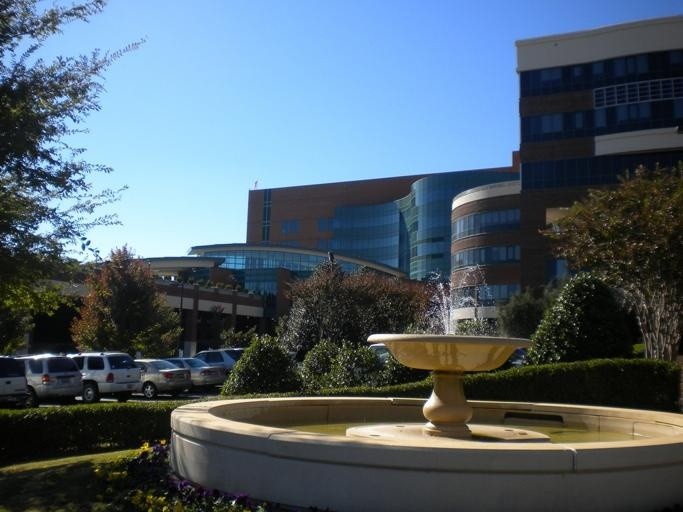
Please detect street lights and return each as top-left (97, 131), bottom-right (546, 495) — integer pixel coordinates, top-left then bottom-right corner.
top-left (170, 277), bottom-right (193, 349)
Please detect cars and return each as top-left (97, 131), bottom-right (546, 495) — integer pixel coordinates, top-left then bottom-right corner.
top-left (0, 348), bottom-right (247, 407)
